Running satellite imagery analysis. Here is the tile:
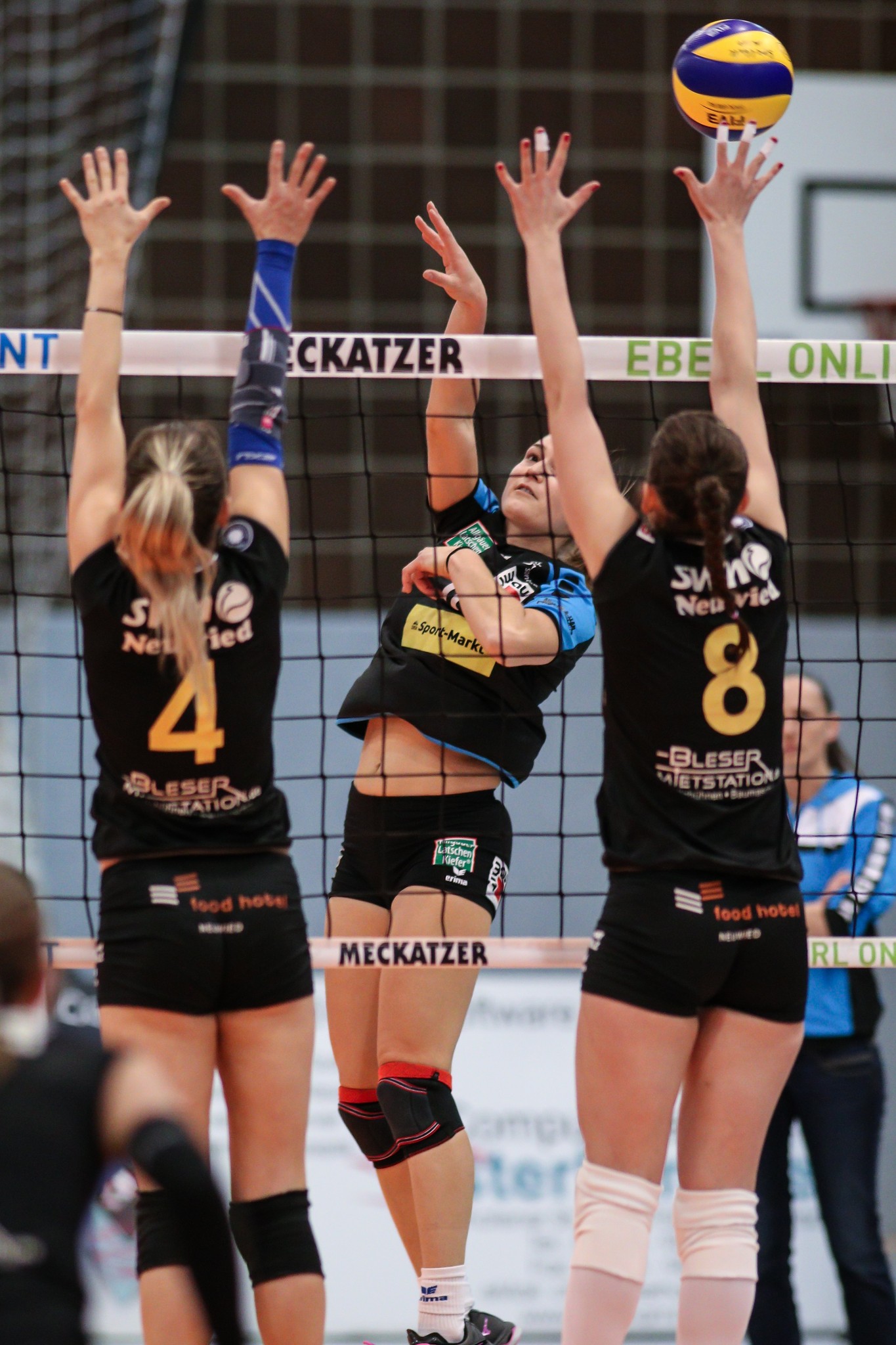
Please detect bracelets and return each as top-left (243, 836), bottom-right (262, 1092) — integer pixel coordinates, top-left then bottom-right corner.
top-left (83, 307), bottom-right (123, 317)
top-left (446, 546), bottom-right (472, 572)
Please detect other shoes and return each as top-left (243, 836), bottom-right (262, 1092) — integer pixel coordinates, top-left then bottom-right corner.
top-left (405, 1308), bottom-right (521, 1345)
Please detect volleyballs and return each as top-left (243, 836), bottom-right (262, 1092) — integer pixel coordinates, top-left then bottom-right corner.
top-left (673, 19), bottom-right (794, 141)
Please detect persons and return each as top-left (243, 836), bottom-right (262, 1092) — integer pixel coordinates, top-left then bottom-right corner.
top-left (748, 669), bottom-right (896, 1345)
top-left (497, 120), bottom-right (810, 1345)
top-left (0, 139), bottom-right (334, 1345)
top-left (324, 202), bottom-right (596, 1345)
